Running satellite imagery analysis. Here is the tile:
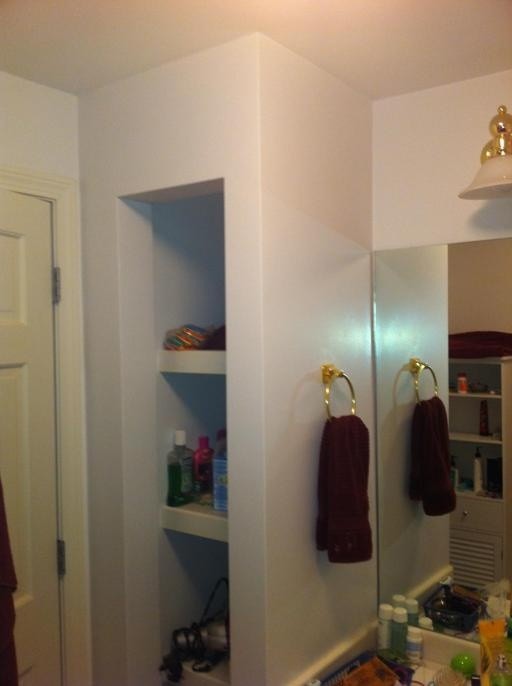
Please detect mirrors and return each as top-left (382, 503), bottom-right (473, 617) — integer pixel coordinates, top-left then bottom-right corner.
top-left (368, 237), bottom-right (511, 651)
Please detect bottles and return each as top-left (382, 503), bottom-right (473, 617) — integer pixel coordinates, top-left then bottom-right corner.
top-left (166, 431), bottom-right (228, 513)
top-left (457, 372), bottom-right (468, 392)
top-left (479, 399), bottom-right (488, 436)
top-left (378, 595), bottom-right (434, 667)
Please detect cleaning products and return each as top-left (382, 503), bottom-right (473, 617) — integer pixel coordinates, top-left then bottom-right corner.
top-left (194, 436), bottom-right (214, 493)
top-left (166, 430), bottom-right (194, 507)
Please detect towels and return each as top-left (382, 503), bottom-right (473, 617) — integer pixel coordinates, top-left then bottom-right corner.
top-left (313, 413), bottom-right (374, 564)
top-left (409, 394), bottom-right (457, 516)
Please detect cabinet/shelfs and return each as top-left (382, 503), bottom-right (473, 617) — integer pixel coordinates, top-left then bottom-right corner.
top-left (109, 179), bottom-right (235, 686)
top-left (445, 357), bottom-right (510, 594)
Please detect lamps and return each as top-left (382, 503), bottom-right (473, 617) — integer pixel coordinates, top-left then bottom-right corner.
top-left (454, 103), bottom-right (512, 200)
